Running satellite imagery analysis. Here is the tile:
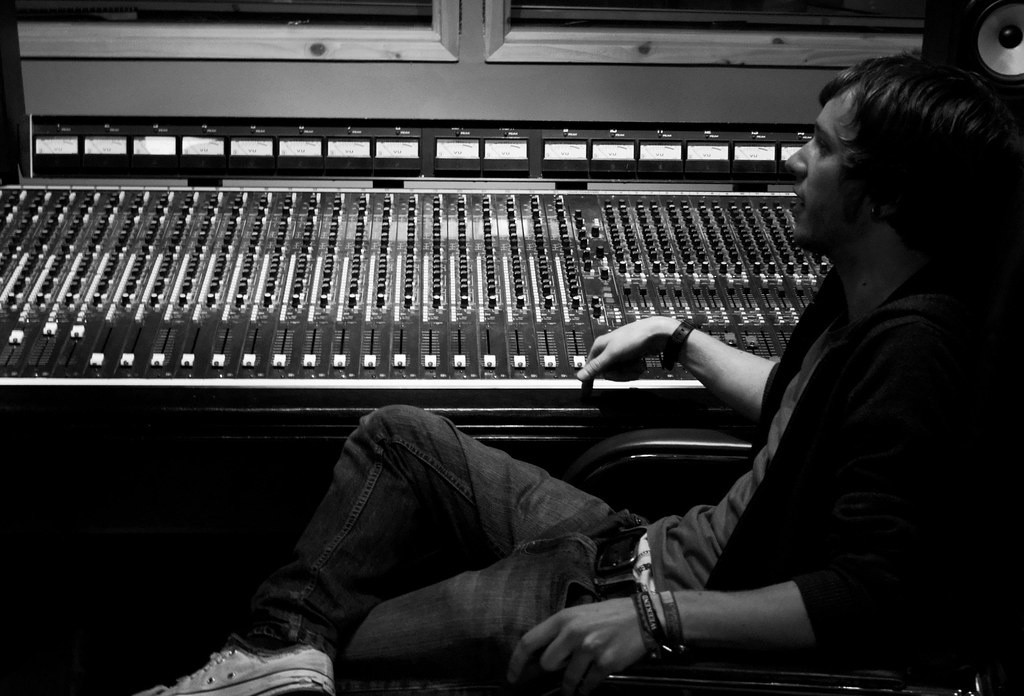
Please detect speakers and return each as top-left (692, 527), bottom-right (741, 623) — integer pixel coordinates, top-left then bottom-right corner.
top-left (922, 0), bottom-right (1024, 137)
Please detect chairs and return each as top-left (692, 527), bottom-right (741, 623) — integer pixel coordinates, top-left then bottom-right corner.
top-left (536, 429), bottom-right (1007, 696)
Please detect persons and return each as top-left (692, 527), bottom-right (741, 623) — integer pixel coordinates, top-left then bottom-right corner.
top-left (142, 51), bottom-right (1024, 696)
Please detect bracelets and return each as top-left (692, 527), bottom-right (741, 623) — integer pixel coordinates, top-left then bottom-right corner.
top-left (631, 591), bottom-right (688, 665)
top-left (663, 320), bottom-right (697, 365)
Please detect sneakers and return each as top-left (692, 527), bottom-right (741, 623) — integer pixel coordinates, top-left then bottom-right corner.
top-left (134, 637), bottom-right (335, 696)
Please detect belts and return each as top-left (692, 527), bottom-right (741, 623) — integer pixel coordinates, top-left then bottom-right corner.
top-left (595, 527), bottom-right (647, 603)
